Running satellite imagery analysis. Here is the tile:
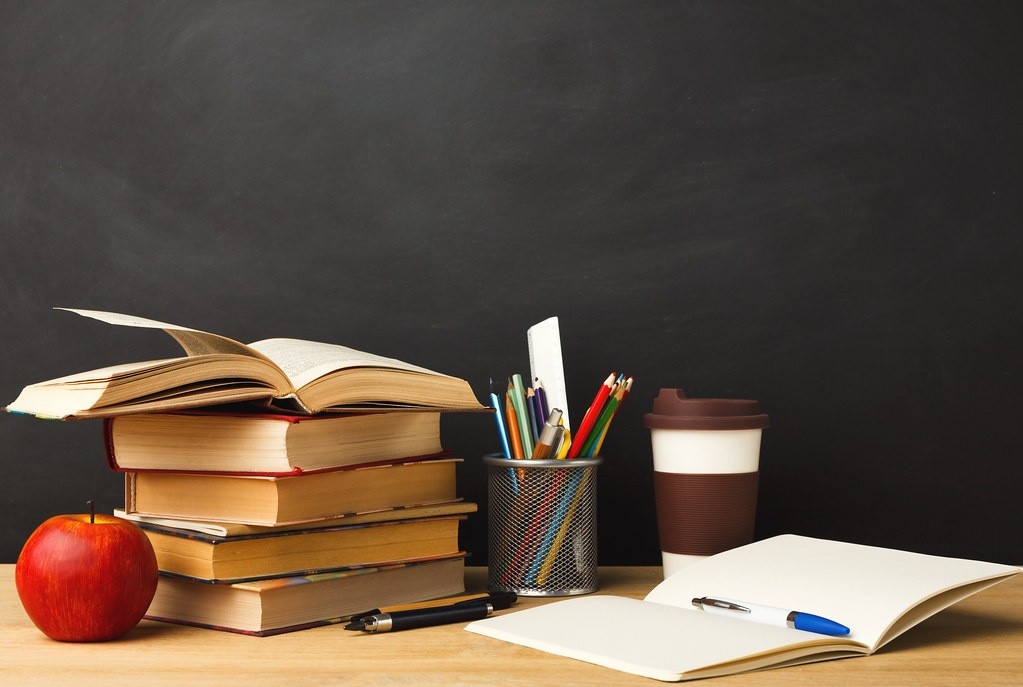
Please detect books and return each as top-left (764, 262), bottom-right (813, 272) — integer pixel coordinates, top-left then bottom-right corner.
top-left (1, 307), bottom-right (498, 421)
top-left (114, 457), bottom-right (477, 638)
top-left (102, 412), bottom-right (454, 475)
top-left (464, 533), bottom-right (1023, 684)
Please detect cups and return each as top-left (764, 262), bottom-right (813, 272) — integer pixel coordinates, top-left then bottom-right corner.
top-left (644, 386), bottom-right (769, 579)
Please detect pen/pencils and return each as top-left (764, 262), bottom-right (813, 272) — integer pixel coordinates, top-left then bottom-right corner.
top-left (488, 373), bottom-right (635, 588)
top-left (343, 592), bottom-right (517, 635)
top-left (692, 597), bottom-right (851, 637)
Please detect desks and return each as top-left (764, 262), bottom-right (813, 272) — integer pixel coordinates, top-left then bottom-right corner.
top-left (0, 562), bottom-right (1023, 687)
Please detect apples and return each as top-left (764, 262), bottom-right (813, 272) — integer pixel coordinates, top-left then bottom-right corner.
top-left (14, 500), bottom-right (159, 643)
